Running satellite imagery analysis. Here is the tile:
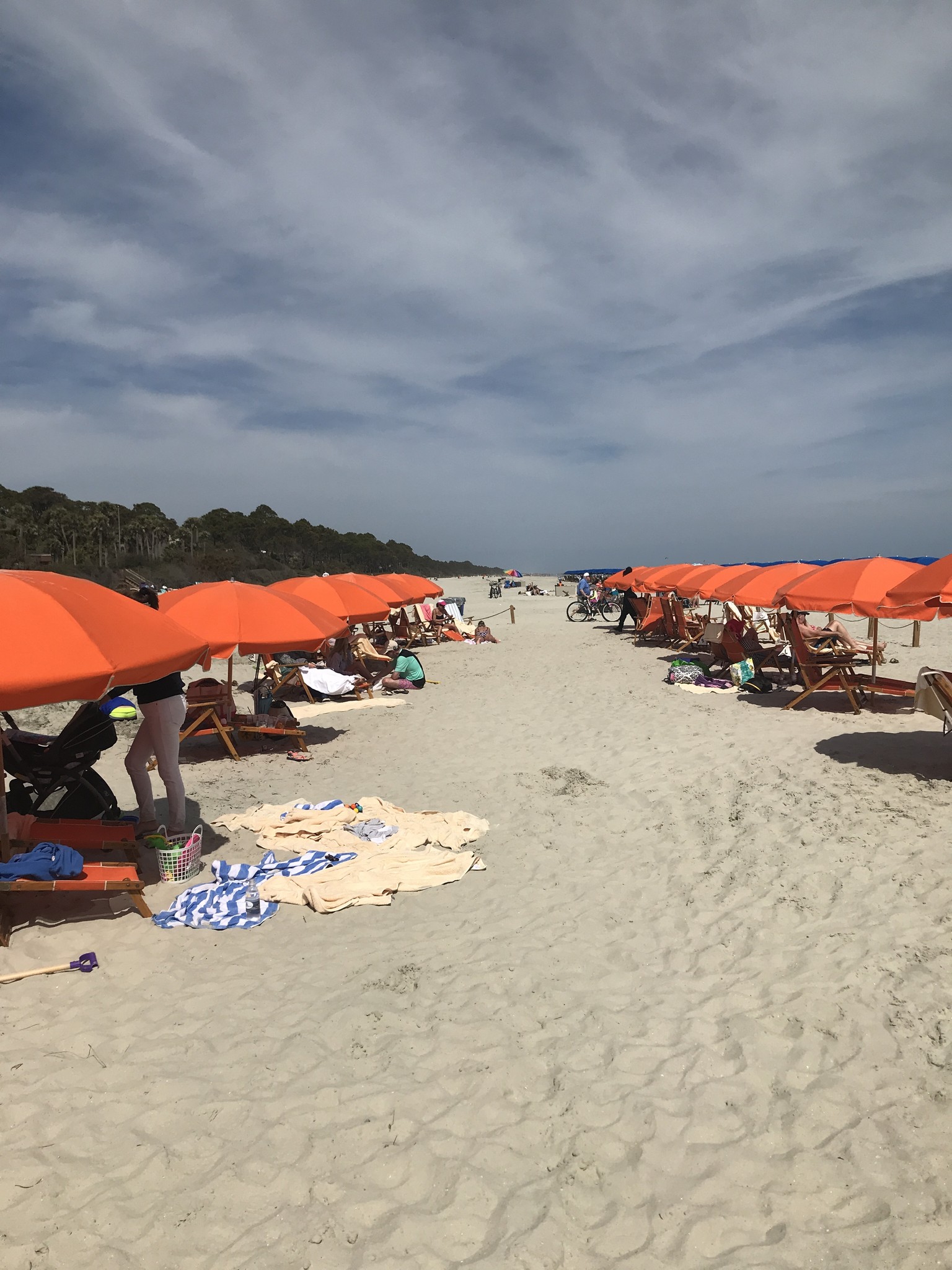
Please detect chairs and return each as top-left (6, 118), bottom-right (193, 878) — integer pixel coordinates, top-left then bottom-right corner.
top-left (230, 713), bottom-right (310, 754)
top-left (443, 603), bottom-right (477, 639)
top-left (249, 651), bottom-right (376, 703)
top-left (913, 664), bottom-right (952, 736)
top-left (30, 816), bottom-right (143, 876)
top-left (412, 602), bottom-right (466, 646)
top-left (620, 590), bottom-right (885, 716)
top-left (387, 606), bottom-right (427, 649)
top-left (319, 639), bottom-right (393, 673)
top-left (144, 698), bottom-right (241, 767)
top-left (361, 621), bottom-right (390, 643)
top-left (1, 857), bottom-right (154, 947)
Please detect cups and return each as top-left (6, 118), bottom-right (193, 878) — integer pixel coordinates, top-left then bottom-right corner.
top-left (258, 714), bottom-right (269, 728)
top-left (268, 716), bottom-right (279, 729)
top-left (276, 715), bottom-right (288, 729)
top-left (772, 676), bottom-right (779, 685)
top-left (246, 714), bottom-right (257, 727)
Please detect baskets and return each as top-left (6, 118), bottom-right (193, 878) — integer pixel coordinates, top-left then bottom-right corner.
top-left (154, 824), bottom-right (204, 884)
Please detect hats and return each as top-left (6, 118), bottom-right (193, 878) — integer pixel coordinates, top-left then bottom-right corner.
top-left (724, 619), bottom-right (745, 640)
top-left (380, 639), bottom-right (398, 655)
top-left (437, 599), bottom-right (446, 605)
top-left (791, 609), bottom-right (810, 618)
top-left (583, 572), bottom-right (592, 577)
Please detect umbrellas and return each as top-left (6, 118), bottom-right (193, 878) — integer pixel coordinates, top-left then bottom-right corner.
top-left (144, 576), bottom-right (352, 747)
top-left (138, 580), bottom-right (201, 596)
top-left (503, 569), bottom-right (523, 585)
top-left (0, 567), bottom-right (213, 870)
top-left (603, 552), bottom-right (952, 707)
top-left (268, 573), bottom-right (446, 649)
top-left (563, 568), bottom-right (630, 576)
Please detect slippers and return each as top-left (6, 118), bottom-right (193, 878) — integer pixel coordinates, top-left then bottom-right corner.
top-left (381, 688), bottom-right (409, 695)
top-left (287, 750), bottom-right (312, 760)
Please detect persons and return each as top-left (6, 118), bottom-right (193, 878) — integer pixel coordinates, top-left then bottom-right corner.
top-left (527, 583), bottom-right (541, 596)
top-left (474, 620), bottom-right (498, 646)
top-left (275, 651), bottom-right (366, 695)
top-left (432, 599), bottom-right (468, 642)
top-left (380, 639), bottom-right (426, 689)
top-left (325, 637), bottom-right (382, 686)
top-left (566, 572), bottom-right (597, 623)
top-left (100, 585), bottom-right (189, 844)
top-left (421, 573), bottom-right (559, 580)
top-left (533, 584), bottom-right (550, 596)
top-left (559, 573), bottom-right (624, 612)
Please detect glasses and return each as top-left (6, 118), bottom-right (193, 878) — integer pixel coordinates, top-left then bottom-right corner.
top-left (440, 605), bottom-right (446, 608)
top-left (803, 614), bottom-right (806, 616)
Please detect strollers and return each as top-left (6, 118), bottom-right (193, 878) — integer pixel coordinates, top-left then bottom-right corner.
top-left (0, 702), bottom-right (122, 821)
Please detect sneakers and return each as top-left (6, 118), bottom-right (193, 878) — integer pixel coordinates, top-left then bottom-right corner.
top-left (566, 614), bottom-right (596, 622)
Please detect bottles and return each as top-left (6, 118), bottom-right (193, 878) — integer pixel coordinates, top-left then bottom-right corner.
top-left (779, 671), bottom-right (786, 686)
top-left (791, 669), bottom-right (798, 686)
top-left (207, 718), bottom-right (227, 728)
top-left (245, 879), bottom-right (261, 922)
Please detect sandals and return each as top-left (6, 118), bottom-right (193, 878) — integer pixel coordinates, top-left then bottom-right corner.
top-left (881, 658), bottom-right (899, 664)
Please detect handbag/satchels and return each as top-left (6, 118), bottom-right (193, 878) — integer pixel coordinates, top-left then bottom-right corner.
top-left (729, 656), bottom-right (773, 693)
top-left (664, 657), bottom-right (712, 684)
top-left (186, 685), bottom-right (236, 723)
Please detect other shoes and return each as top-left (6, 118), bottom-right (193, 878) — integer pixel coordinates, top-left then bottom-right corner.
top-left (613, 626), bottom-right (636, 632)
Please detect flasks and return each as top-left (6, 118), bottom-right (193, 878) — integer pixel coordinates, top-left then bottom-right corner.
top-left (559, 581), bottom-right (562, 586)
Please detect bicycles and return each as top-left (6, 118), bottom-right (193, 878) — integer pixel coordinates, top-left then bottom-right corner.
top-left (567, 588), bottom-right (622, 623)
top-left (492, 581), bottom-right (503, 598)
top-left (486, 579), bottom-right (497, 598)
